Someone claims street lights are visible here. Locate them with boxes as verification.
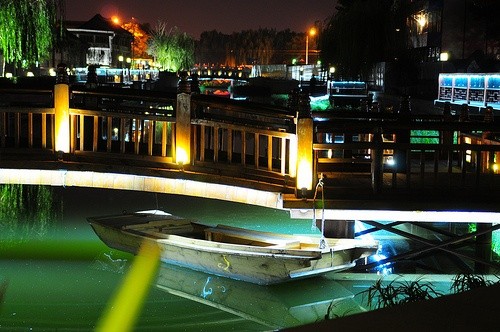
[304,27,316,64]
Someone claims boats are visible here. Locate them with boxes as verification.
[84,209,378,286]
